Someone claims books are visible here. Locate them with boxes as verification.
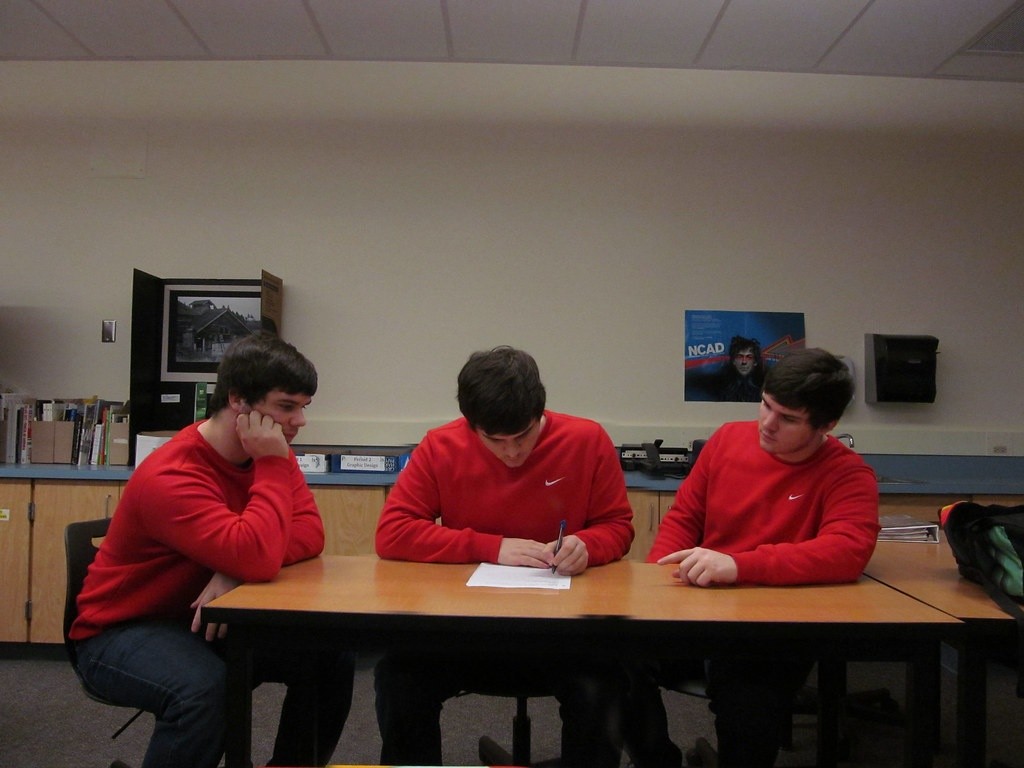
[0,393,132,470]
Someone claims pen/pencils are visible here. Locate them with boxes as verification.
[552,520,567,575]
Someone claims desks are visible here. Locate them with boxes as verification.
[197,554,966,768]
[864,529,1024,768]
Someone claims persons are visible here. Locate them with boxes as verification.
[374,345,638,766]
[615,343,883,768]
[68,328,359,768]
[710,335,777,403]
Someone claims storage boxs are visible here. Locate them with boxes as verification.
[134,431,179,469]
[54,421,75,464]
[330,449,407,475]
[295,450,331,473]
[31,420,54,464]
[108,422,129,466]
[399,449,413,471]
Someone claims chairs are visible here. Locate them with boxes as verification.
[660,670,719,768]
[460,670,562,768]
[63,517,264,768]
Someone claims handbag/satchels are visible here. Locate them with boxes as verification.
[937,501,1024,626]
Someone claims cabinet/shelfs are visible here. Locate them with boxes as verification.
[309,484,441,556]
[26,479,127,660]
[0,477,33,660]
[625,487,677,559]
